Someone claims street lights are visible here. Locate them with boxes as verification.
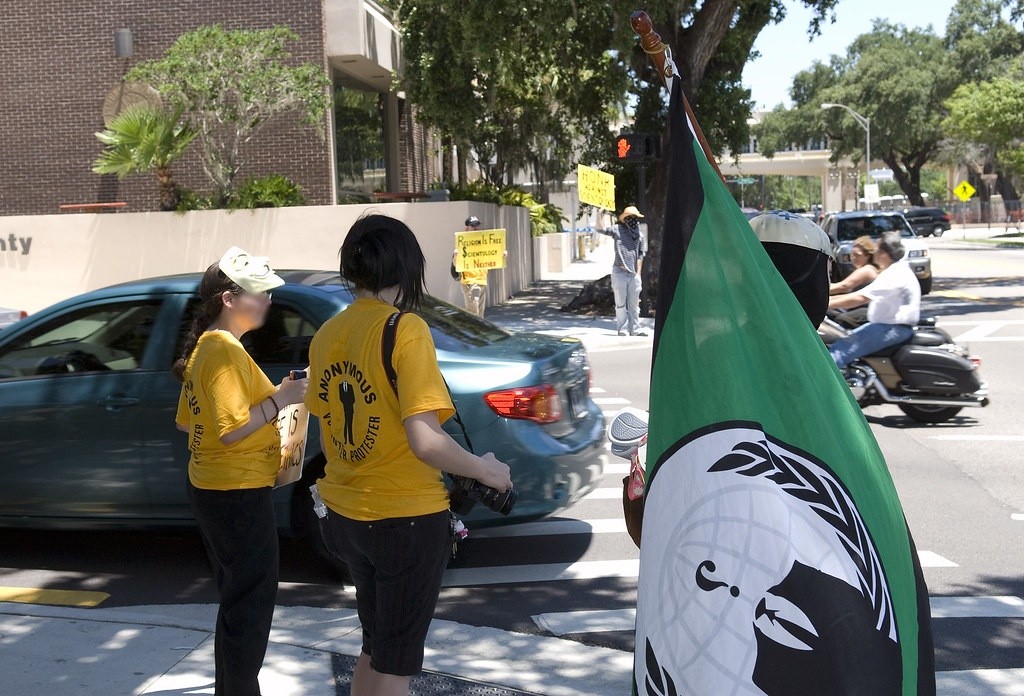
[822,103,873,210]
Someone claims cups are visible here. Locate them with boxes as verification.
[290,370,307,379]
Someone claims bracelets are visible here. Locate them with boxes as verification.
[266,395,279,418]
[260,403,269,425]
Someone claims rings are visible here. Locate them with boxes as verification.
[508,482,513,491]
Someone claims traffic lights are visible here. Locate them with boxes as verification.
[612,133,643,165]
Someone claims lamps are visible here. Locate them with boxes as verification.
[114,29,133,58]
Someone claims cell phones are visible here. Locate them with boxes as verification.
[290,370,307,381]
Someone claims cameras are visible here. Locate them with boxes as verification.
[449,475,520,515]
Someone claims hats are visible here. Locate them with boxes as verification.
[465,216,481,227]
[619,207,646,222]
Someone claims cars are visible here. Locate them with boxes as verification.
[740,208,759,222]
[897,208,951,238]
[0,269,608,579]
[794,213,825,227]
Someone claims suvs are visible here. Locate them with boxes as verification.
[821,210,933,296]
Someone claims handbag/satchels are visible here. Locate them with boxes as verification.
[451,263,463,282]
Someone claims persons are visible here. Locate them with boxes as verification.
[596,206,648,337]
[302,214,513,696]
[825,237,879,329]
[175,249,310,696]
[819,231,921,368]
[453,216,507,321]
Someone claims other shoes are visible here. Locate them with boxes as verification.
[619,330,648,338]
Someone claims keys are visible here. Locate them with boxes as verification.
[451,513,462,557]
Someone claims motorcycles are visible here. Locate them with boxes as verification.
[825,307,953,347]
[817,315,992,423]
